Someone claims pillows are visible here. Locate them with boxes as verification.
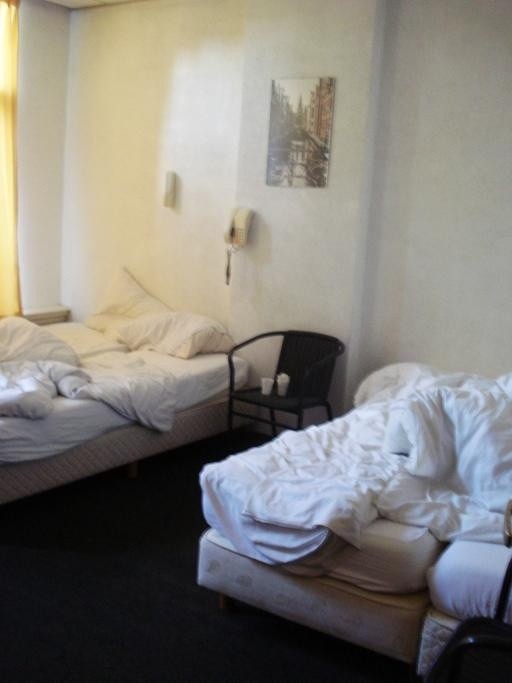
[87,266,235,359]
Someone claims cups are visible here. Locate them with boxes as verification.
[261,377,274,395]
[276,373,290,396]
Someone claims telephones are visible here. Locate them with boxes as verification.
[222,207,254,286]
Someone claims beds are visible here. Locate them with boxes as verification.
[0,265,254,506]
[197,362,512,678]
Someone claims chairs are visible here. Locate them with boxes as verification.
[228,331,346,438]
